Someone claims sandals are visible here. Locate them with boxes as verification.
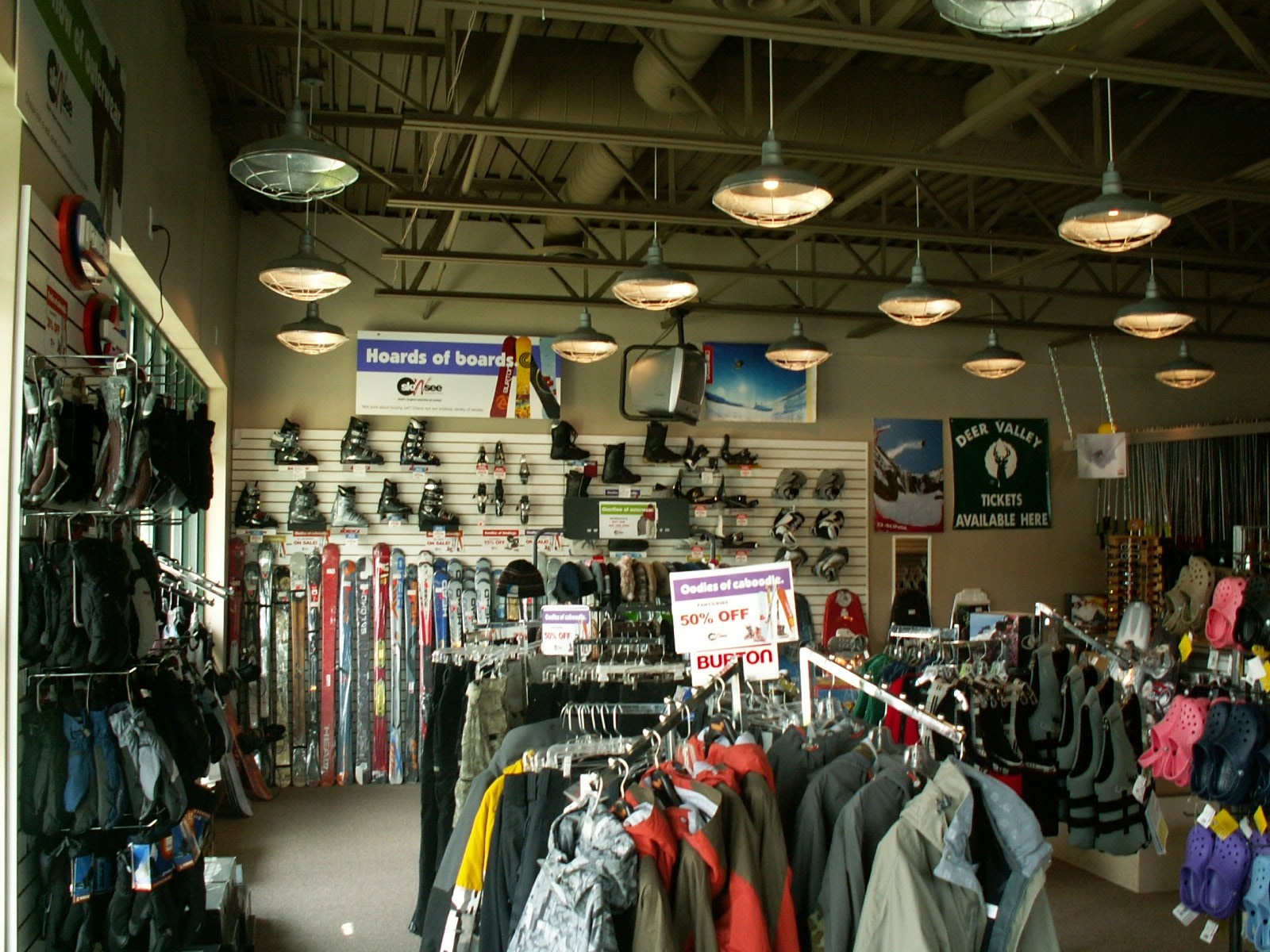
[1137,558,1270,952]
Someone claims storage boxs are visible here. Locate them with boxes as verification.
[203,856,256,952]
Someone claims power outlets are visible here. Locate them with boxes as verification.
[148,203,157,241]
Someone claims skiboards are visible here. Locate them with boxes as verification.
[224,535,561,788]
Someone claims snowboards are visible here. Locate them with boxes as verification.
[489,336,560,418]
[209,659,273,817]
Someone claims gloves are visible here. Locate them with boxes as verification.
[21,364,226,952]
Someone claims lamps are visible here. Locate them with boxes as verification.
[259,202,351,302]
[765,230,830,372]
[1057,78,1171,251]
[1154,261,1217,390]
[879,169,961,326]
[611,148,699,311]
[1114,191,1196,339]
[551,233,619,363]
[230,0,361,202]
[961,231,1026,380]
[276,198,350,355]
[713,39,834,227]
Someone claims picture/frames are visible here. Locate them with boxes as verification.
[890,535,933,628]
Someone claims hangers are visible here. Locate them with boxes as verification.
[158,570,198,612]
[433,604,1270,842]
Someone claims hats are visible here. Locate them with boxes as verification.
[497,560,544,598]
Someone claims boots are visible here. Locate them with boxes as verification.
[331,485,369,527]
[270,418,318,465]
[551,420,589,460]
[418,480,459,532]
[234,481,278,528]
[771,467,850,583]
[399,415,441,466]
[602,444,641,484]
[565,470,592,498]
[341,417,386,464]
[644,421,680,463]
[286,482,328,531]
[377,480,411,514]
